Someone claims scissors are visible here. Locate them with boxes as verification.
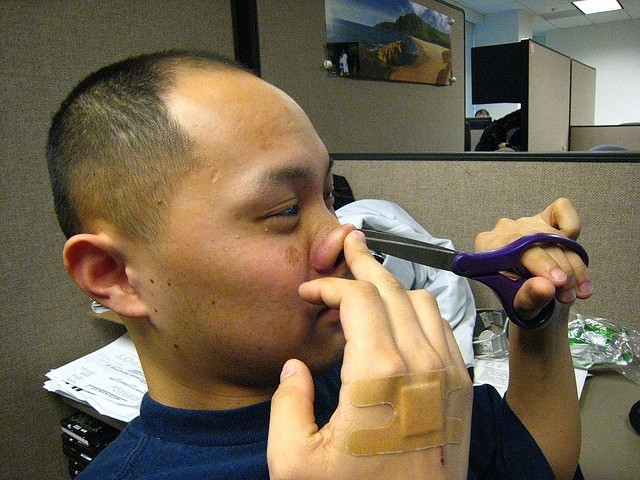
[357,229,589,330]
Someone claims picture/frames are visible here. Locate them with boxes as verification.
[323,0,456,87]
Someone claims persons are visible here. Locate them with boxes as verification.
[45,49,594,479]
[343,49,348,74]
[474,109,489,117]
[339,53,343,76]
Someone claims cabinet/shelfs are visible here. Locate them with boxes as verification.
[43,310,126,478]
[471,39,596,153]
